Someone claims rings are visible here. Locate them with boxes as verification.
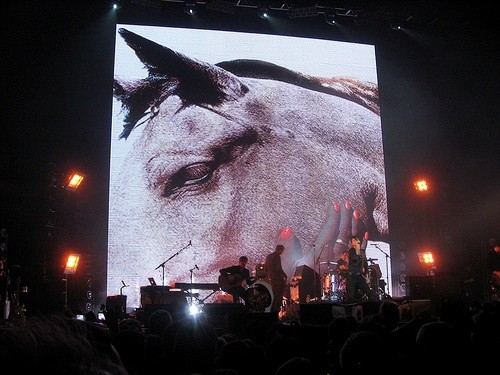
[336,239,347,245]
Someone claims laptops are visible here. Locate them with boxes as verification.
[148,278,156,285]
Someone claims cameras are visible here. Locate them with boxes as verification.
[77,315,86,321]
[98,313,106,320]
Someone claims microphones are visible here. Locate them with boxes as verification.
[195,265,199,270]
[370,244,377,245]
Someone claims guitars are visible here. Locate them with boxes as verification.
[219,272,259,291]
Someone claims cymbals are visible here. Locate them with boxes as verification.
[367,259,378,261]
[319,261,338,265]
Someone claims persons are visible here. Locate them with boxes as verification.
[219,256,251,303]
[337,251,349,271]
[278,200,369,285]
[346,236,369,303]
[264,244,287,312]
[0,300,500,375]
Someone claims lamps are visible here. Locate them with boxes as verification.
[58,250,81,272]
[62,166,84,189]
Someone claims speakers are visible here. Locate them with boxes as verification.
[105,296,126,330]
[205,303,243,336]
[289,264,322,302]
[228,312,278,340]
[294,303,333,325]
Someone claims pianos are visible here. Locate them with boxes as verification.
[170,282,219,316]
